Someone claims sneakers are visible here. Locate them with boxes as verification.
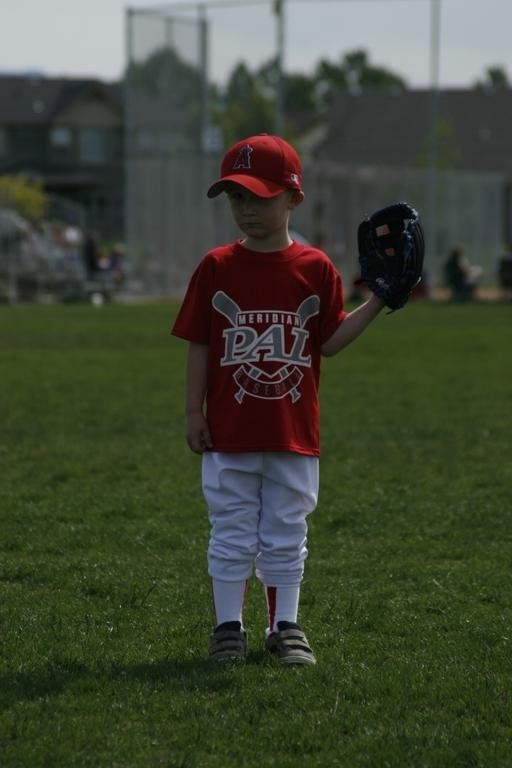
[209,620,248,672]
[264,620,316,667]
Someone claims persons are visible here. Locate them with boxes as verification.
[444,245,474,306]
[170,132,427,670]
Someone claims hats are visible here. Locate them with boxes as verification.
[206,132,303,199]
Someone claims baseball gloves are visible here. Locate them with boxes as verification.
[354,202,425,315]
